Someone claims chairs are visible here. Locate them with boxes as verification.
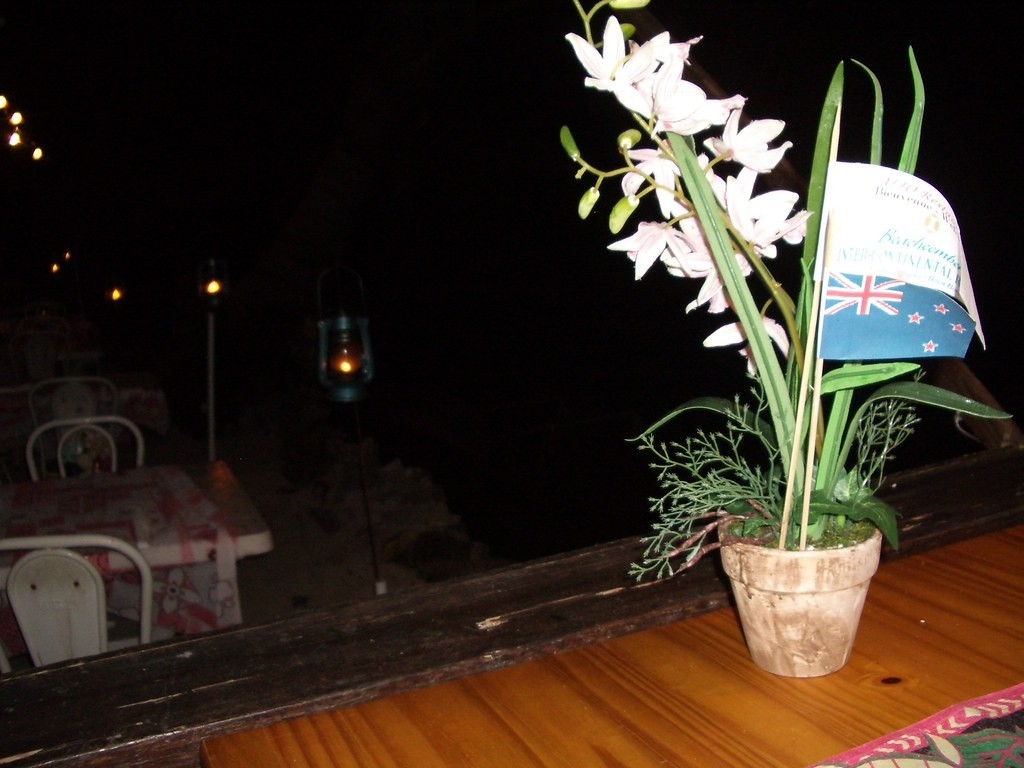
[0,532,152,673]
[7,300,145,481]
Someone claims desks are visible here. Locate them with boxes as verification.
[0,371,170,475]
[0,460,272,636]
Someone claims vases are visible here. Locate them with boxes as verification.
[717,508,882,677]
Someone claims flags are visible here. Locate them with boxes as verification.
[813,162,986,359]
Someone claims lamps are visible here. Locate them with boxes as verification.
[316,309,382,600]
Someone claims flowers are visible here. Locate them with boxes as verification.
[560,0,1014,583]
